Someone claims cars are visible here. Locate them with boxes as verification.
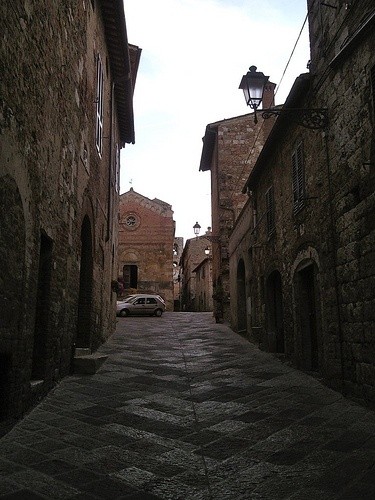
[115,295,165,317]
[116,294,147,305]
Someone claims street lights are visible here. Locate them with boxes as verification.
[237,65,348,398]
[194,222,222,272]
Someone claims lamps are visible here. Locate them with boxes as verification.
[193,222,219,243]
[239,66,327,129]
[204,246,210,254]
